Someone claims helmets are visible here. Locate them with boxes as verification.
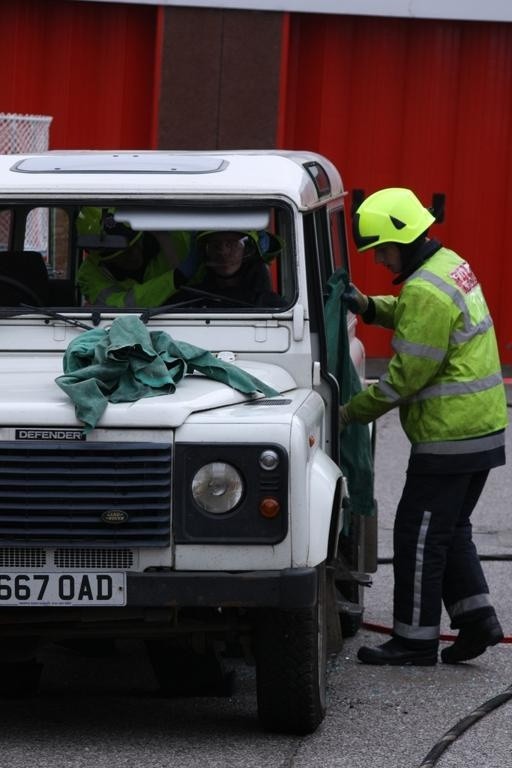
[352,187,437,254]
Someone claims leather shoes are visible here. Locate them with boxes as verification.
[441,614,504,664]
[357,636,439,664]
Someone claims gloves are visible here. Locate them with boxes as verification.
[343,282,368,315]
[339,405,351,436]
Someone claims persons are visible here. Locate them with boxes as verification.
[77,204,289,308]
[339,188,509,667]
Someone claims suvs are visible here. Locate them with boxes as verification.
[0,150,380,736]
